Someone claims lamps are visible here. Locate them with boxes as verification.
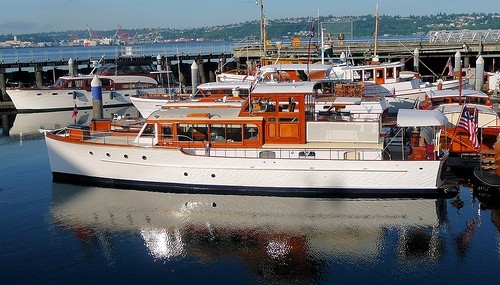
[248,128,256,137]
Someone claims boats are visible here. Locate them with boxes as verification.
[0,71,182,137]
[37,75,450,189]
[196,0,500,160]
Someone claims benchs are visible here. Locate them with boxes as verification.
[91,118,111,132]
[67,124,91,140]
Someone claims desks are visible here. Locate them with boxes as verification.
[192,131,205,140]
